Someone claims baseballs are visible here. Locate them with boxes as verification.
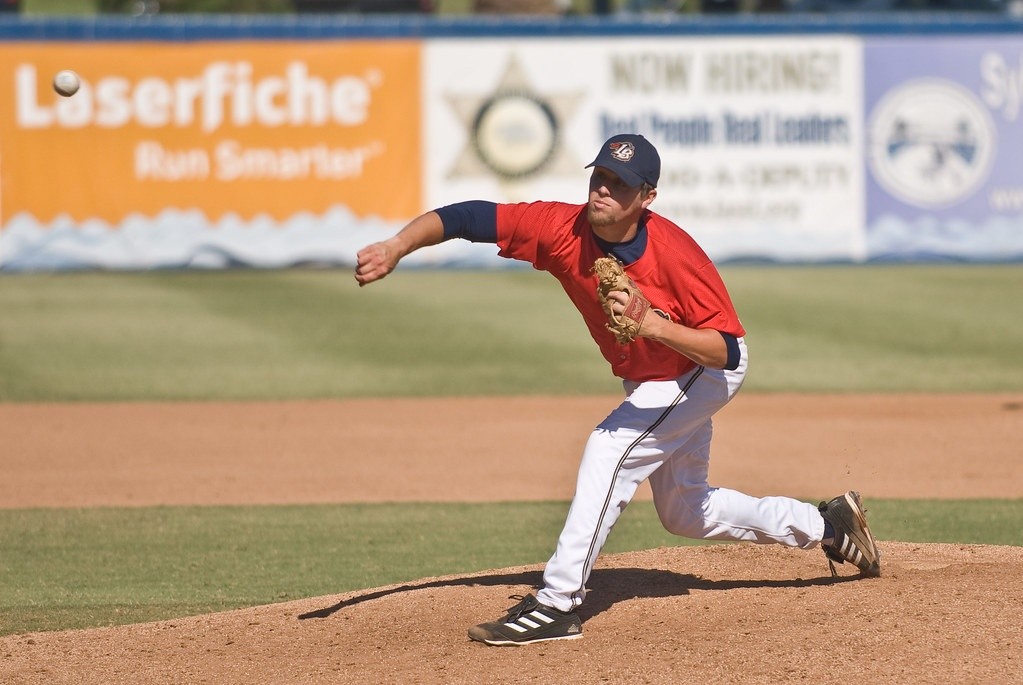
[53,70,80,97]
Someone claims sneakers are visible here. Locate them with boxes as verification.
[467,592,585,646]
[818,489,881,577]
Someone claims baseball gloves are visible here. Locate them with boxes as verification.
[588,252,651,345]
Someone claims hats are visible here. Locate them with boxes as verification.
[585,133,661,188]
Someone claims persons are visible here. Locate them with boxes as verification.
[354,132,879,647]
